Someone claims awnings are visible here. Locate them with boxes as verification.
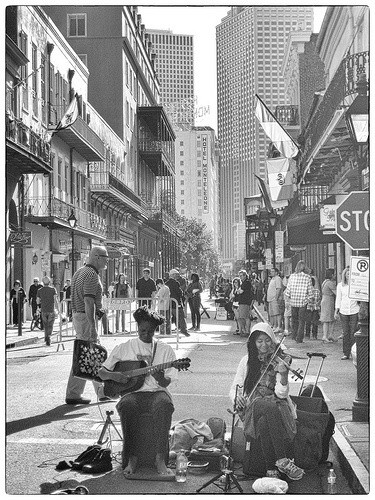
[286,212,342,244]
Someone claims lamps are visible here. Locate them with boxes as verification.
[33,252,38,264]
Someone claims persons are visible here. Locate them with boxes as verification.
[112,274,129,333]
[136,268,202,337]
[334,268,361,360]
[63,279,73,322]
[267,265,321,339]
[283,260,315,343]
[229,270,265,337]
[209,273,232,299]
[28,278,43,330]
[230,322,304,480]
[10,279,26,325]
[65,245,110,404]
[98,304,178,476]
[319,268,338,343]
[102,309,112,334]
[36,277,56,346]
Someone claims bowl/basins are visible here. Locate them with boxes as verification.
[187,461,209,474]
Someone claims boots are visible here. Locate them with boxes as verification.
[71,444,102,470]
[83,448,113,473]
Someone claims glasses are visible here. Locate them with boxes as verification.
[33,280,38,282]
[94,253,110,258]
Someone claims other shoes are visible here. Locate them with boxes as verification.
[14,322,23,327]
[66,397,92,404]
[45,337,50,346]
[181,326,201,337]
[291,334,318,343]
[104,329,129,334]
[271,325,291,337]
[341,356,348,360]
[233,329,249,338]
[99,396,111,401]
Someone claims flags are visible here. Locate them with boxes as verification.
[255,100,298,159]
[62,96,80,129]
[266,158,298,200]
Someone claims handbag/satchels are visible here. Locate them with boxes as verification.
[71,338,108,383]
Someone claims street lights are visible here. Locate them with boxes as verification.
[67,208,77,276]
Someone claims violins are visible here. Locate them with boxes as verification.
[264,343,304,381]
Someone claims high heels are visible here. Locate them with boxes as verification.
[321,336,339,345]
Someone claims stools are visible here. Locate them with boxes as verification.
[243,437,294,477]
[122,413,170,468]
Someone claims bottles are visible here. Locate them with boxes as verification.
[175,449,188,483]
[327,469,338,494]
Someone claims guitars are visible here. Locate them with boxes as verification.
[103,356,191,399]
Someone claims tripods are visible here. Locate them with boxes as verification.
[98,411,122,444]
[194,383,244,494]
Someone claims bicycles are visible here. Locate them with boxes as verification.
[30,303,44,331]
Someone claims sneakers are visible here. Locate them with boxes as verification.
[275,458,306,480]
[267,470,282,480]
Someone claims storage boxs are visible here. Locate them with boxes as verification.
[188,440,227,474]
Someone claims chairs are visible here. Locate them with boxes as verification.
[200,304,210,318]
[214,285,232,320]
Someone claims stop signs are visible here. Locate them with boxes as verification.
[333,191,369,251]
